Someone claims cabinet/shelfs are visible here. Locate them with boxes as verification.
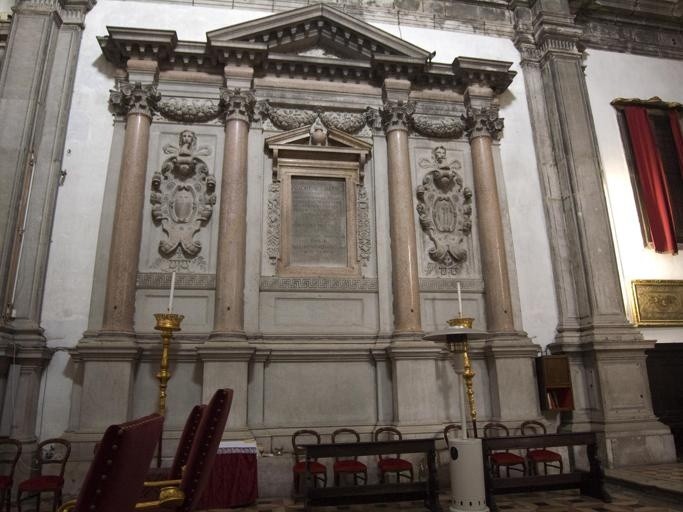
[534,354,574,411]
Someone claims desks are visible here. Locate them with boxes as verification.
[479,431,615,511]
[201,439,258,508]
[297,435,446,512]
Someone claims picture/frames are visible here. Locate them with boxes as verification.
[629,278,683,328]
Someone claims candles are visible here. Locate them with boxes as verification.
[167,271,175,311]
[456,280,463,318]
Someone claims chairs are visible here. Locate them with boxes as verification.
[442,422,564,477]
[59,385,237,511]
[288,426,415,508]
[0,430,69,511]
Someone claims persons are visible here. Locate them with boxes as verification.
[178,129,200,153]
[430,146,447,166]
